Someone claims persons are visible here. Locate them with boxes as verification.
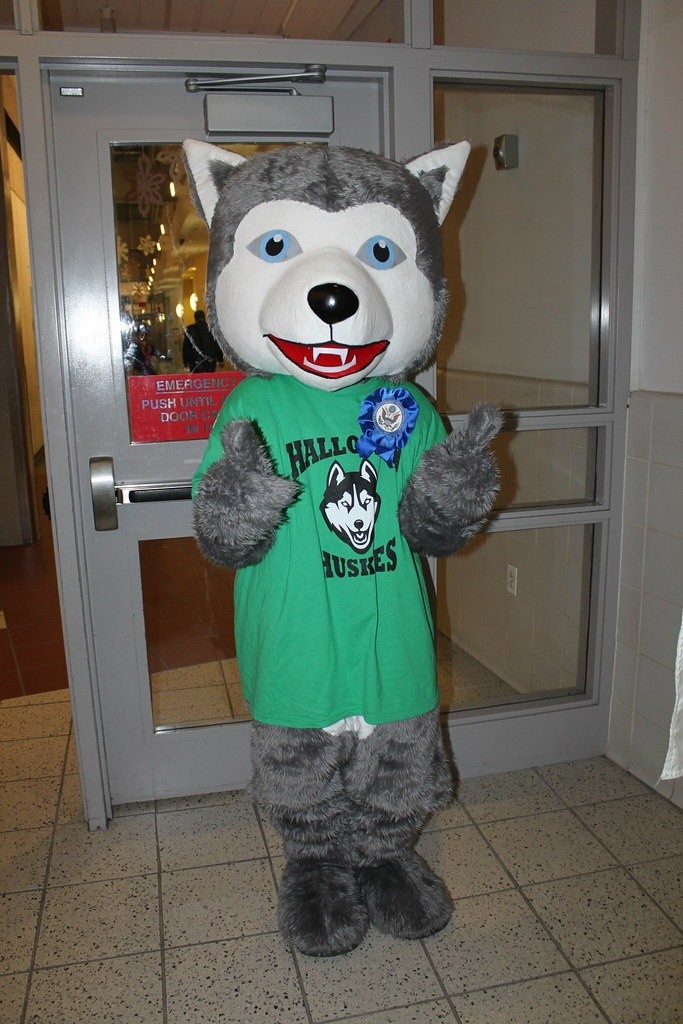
[182,310,224,372]
[123,322,159,376]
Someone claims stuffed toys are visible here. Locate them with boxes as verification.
[180,137,509,956]
